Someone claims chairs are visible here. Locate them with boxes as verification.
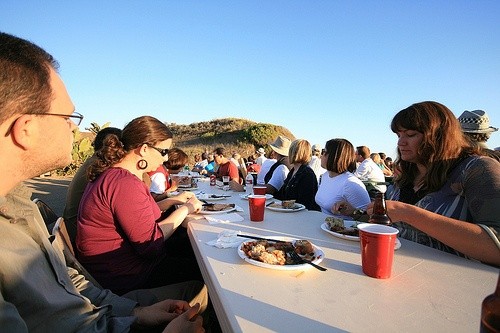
[33,197,105,288]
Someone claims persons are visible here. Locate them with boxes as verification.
[330,100,500,266]
[74,116,203,284]
[0,31,205,332]
[63,126,394,247]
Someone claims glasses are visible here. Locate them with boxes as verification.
[6,112,83,136]
[149,145,168,157]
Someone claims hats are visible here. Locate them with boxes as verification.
[269,135,291,156]
[256,148,266,154]
[457,110,498,134]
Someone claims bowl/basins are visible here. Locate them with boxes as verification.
[253,188,267,195]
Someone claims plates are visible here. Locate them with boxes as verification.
[197,194,231,201]
[321,220,367,241]
[176,184,199,190]
[197,203,236,214]
[265,201,307,212]
[237,236,325,270]
[241,194,274,199]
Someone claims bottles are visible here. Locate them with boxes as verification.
[369,192,393,227]
[479,271,500,333]
[210,173,217,186]
[245,172,255,196]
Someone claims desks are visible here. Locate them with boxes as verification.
[171,176,309,228]
[187,210,500,333]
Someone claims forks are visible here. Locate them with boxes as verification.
[290,250,328,271]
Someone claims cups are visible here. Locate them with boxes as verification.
[247,195,267,221]
[357,223,400,279]
[222,176,230,185]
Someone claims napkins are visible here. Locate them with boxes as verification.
[204,229,250,249]
[202,211,244,225]
